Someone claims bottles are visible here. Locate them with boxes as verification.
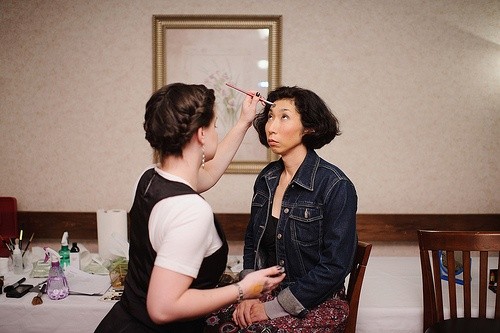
[490,272,496,282]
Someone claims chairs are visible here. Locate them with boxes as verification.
[343,241,372,333]
[417,229,500,333]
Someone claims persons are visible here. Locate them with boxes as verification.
[206,86,358,333]
[93,84,286,333]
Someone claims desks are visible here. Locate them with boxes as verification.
[0,255,243,333]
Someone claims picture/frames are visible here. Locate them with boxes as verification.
[151,12,284,174]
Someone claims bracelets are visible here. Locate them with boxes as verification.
[233,283,243,303]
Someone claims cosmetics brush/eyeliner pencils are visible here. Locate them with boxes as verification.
[2,230,46,304]
[225,84,275,107]
